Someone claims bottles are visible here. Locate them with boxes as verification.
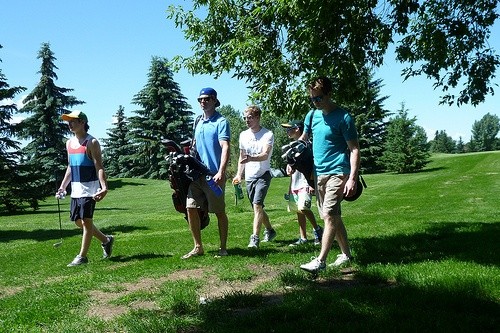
[234,180,244,199]
[205,175,223,196]
[59,188,65,202]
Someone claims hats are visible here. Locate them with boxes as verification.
[61,110,88,122]
[281,120,305,128]
[199,88,220,108]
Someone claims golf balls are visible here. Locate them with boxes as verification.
[55,190,67,200]
[92,188,101,198]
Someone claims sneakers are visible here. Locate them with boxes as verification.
[327,252,354,267]
[289,238,308,247]
[261,228,276,243]
[67,255,88,269]
[248,234,261,250]
[300,257,327,274]
[313,226,324,246]
[101,235,114,258]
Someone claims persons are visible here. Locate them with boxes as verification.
[282,121,324,246]
[55,111,114,268]
[181,88,231,259]
[232,105,276,249]
[296,76,360,274]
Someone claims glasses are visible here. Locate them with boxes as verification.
[310,94,326,102]
[243,115,257,121]
[198,97,213,102]
[286,127,299,131]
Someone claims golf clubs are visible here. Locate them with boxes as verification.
[53,169,63,247]
[160,139,197,176]
[280,139,306,161]
